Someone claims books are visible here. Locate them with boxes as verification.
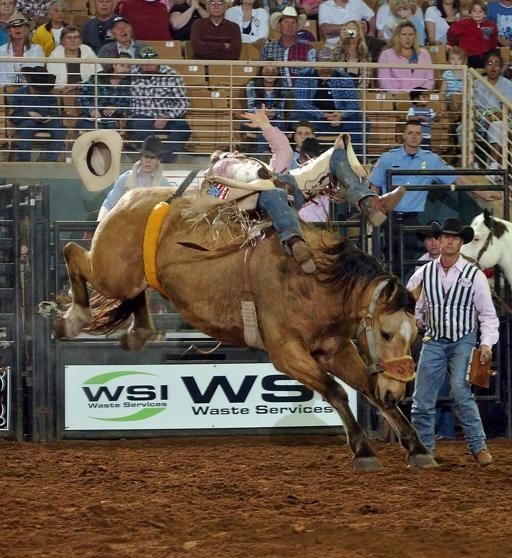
[466,347,494,389]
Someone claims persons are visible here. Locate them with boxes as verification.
[407,218,499,469]
[416,221,459,441]
[293,46,371,165]
[0,0,16,45]
[318,20,370,90]
[116,46,192,163]
[376,1,425,47]
[241,56,292,155]
[318,0,387,63]
[255,7,316,119]
[447,2,502,69]
[440,48,468,97]
[0,11,46,86]
[113,0,169,41]
[368,121,503,286]
[378,21,435,93]
[204,106,405,274]
[46,26,104,92]
[81,1,120,55]
[9,65,67,162]
[32,1,71,57]
[425,1,465,46]
[225,1,269,44]
[393,2,427,47]
[406,86,436,148]
[191,0,242,61]
[98,17,148,71]
[74,53,132,132]
[486,0,511,40]
[97,135,169,222]
[169,0,209,40]
[450,49,512,180]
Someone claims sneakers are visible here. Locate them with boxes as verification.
[486,173,501,182]
[474,161,479,167]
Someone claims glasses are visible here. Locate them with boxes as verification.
[488,59,499,66]
[206,0,227,6]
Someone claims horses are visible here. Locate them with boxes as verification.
[458,208,511,287]
[51,188,442,473]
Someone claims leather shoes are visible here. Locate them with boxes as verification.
[476,450,495,468]
[358,186,406,228]
[288,237,318,275]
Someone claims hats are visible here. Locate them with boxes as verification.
[432,217,476,245]
[136,135,166,158]
[316,46,336,61]
[138,47,161,58]
[21,64,58,92]
[5,10,28,28]
[106,13,129,29]
[268,7,310,30]
[415,219,442,238]
